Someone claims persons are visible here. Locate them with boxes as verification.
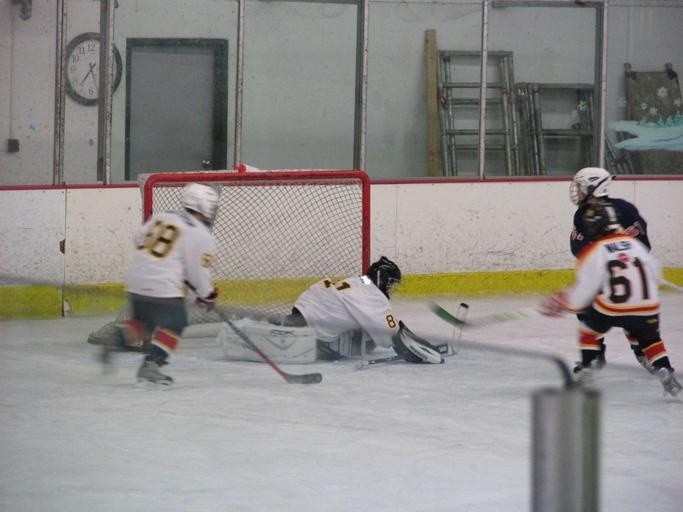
[565,167,662,376]
[540,200,680,400]
[83,180,219,389]
[216,250,458,365]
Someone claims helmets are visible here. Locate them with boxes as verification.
[569,167,613,206]
[366,256,401,298]
[180,181,221,226]
[575,206,610,239]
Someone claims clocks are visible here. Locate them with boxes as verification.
[64,32,122,105]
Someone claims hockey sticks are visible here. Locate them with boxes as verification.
[353,302,468,369]
[429,303,540,328]
[182,282,322,384]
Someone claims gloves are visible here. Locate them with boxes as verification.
[195,288,219,312]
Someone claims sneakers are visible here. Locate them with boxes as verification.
[136,360,172,387]
[572,355,606,375]
[660,372,682,399]
